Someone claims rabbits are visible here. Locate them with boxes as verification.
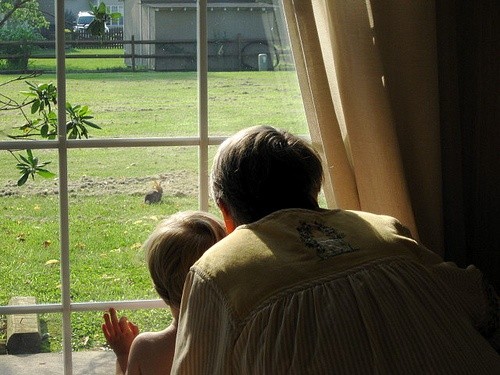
[144,180,163,206]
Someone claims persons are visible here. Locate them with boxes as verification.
[169,122,500,375]
[102,210,229,375]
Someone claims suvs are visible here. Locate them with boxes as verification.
[73,11,110,39]
